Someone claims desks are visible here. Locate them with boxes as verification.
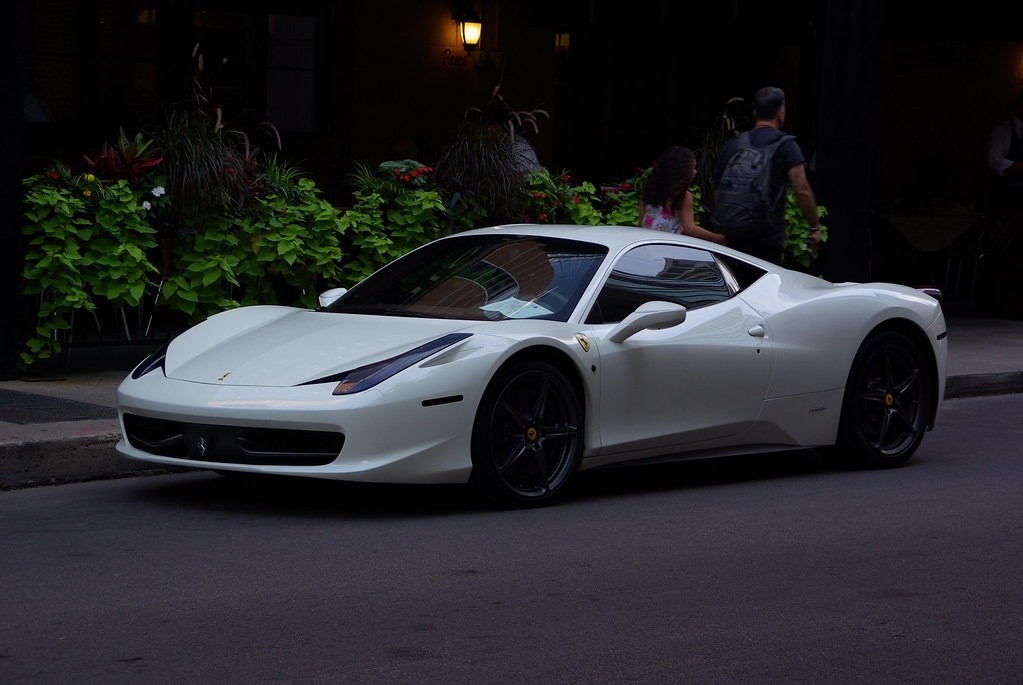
[881,210,978,294]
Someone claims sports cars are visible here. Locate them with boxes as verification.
[115,222,949,509]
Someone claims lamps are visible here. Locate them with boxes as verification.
[451,5,483,57]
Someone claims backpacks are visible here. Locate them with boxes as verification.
[710,132,796,233]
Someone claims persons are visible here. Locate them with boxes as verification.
[714,87,821,268]
[392,124,540,226]
[638,146,726,245]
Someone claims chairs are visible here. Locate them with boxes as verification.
[944,227,992,297]
